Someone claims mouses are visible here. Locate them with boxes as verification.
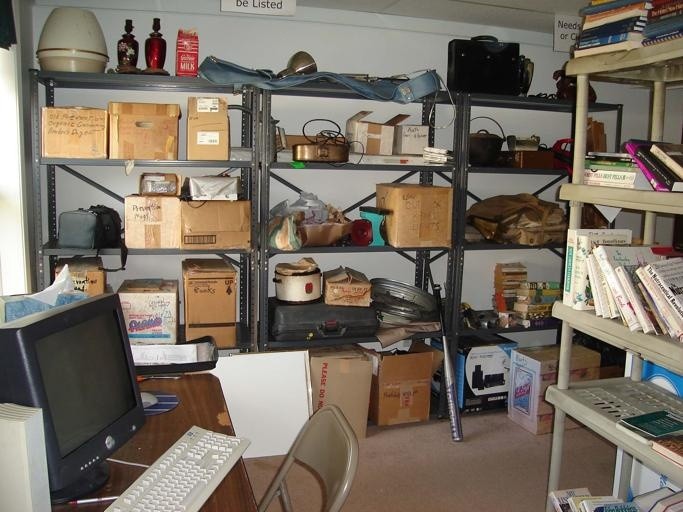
[140,391,158,408]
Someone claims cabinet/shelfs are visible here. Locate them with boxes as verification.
[544,36,682,512]
[28,67,260,354]
[260,79,463,422]
[446,92,624,418]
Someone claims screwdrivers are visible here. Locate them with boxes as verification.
[136,374,181,382]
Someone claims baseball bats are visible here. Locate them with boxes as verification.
[432,284,463,440]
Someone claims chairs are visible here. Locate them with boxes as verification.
[258,405,359,512]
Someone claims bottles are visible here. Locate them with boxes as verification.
[115,19,139,69]
[144,17,166,70]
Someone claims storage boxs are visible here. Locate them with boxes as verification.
[375,183,454,248]
[507,343,601,436]
[322,266,371,308]
[41,104,108,160]
[343,110,411,156]
[420,333,519,417]
[124,173,252,251]
[362,341,444,426]
[187,96,231,161]
[393,124,430,156]
[270,345,373,441]
[54,258,238,349]
[108,101,182,161]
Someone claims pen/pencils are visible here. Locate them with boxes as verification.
[67,496,119,505]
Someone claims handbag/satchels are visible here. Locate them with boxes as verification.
[448,35,520,96]
[58,207,120,249]
[466,191,566,244]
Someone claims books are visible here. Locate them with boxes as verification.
[563,229,683,341]
[583,139,683,193]
[549,487,683,512]
[617,407,683,466]
[493,262,563,328]
[574,0,683,57]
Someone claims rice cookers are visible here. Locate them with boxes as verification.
[273,269,323,305]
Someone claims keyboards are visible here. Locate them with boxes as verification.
[104,425,251,512]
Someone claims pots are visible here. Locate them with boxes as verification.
[292,142,351,163]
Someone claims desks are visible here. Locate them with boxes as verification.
[50,372,261,512]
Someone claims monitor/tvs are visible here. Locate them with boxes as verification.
[0,294,148,504]
